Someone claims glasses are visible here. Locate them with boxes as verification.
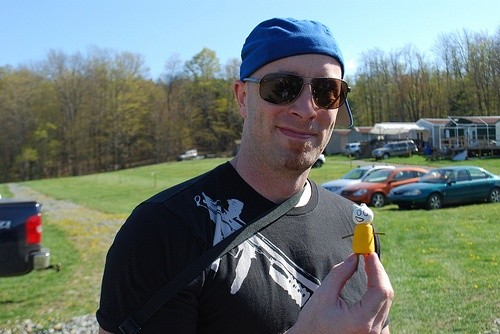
[242,73,352,110]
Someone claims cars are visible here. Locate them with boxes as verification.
[387,166,500,210]
[320,165,403,195]
[0,201,50,278]
[312,154,326,168]
[341,166,448,208]
[177,149,198,161]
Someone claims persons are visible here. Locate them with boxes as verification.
[95,16,395,334]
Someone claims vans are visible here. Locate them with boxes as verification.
[344,142,361,155]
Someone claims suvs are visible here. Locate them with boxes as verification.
[371,140,418,160]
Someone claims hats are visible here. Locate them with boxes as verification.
[240,18,345,82]
